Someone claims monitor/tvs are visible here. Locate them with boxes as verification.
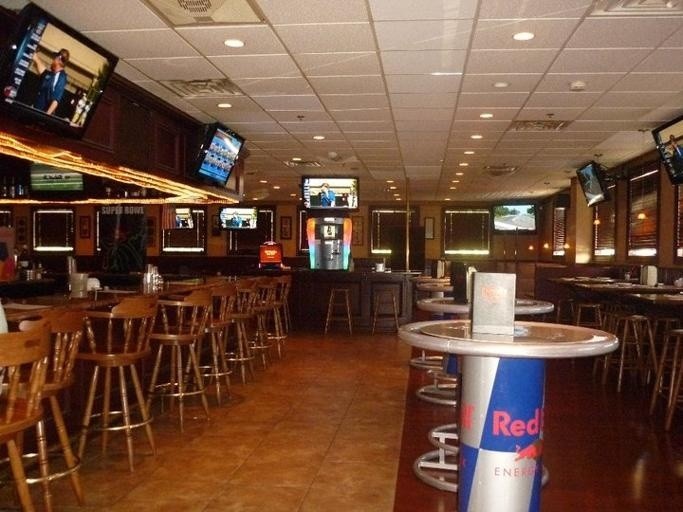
[28,160,86,199]
[175,207,195,230]
[302,175,359,212]
[490,203,537,235]
[191,122,246,186]
[0,1,120,142]
[575,160,610,208]
[218,206,259,231]
[650,115,683,186]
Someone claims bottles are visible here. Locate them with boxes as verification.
[1,171,25,197]
[142,263,163,286]
[103,180,146,197]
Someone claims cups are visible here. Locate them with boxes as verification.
[375,262,385,273]
[68,273,89,298]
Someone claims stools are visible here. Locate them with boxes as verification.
[322,286,354,336]
[370,287,399,336]
